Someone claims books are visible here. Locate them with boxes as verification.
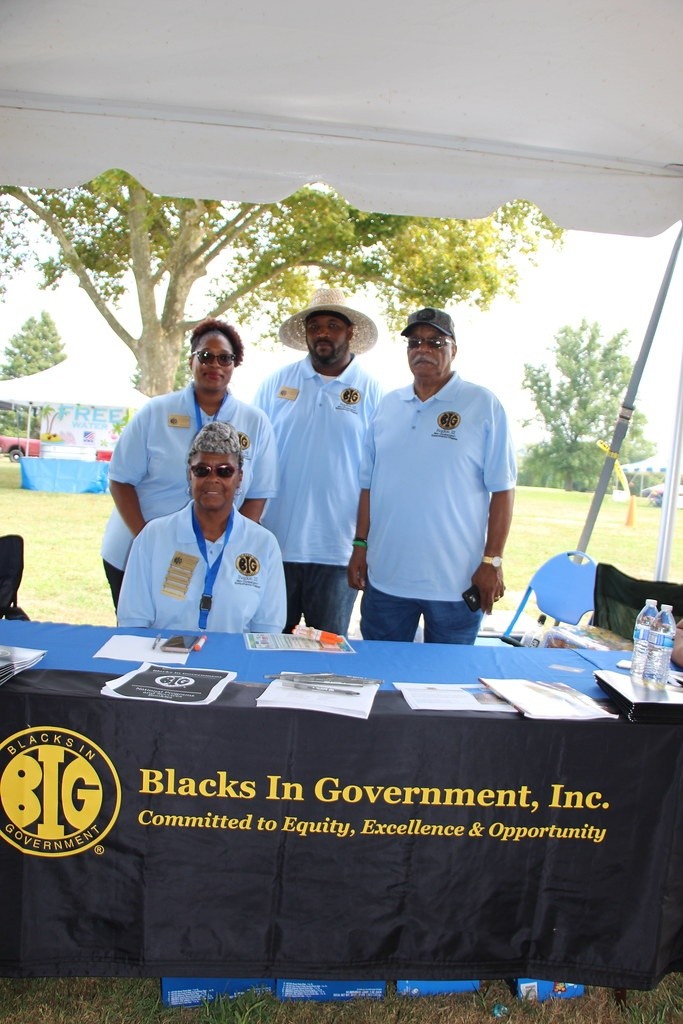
[159,633,200,653]
[100,662,237,705]
[477,678,619,722]
[593,670,683,725]
[0,644,48,685]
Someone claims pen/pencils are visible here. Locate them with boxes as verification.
[152,633,162,649]
[264,672,384,688]
[282,682,360,696]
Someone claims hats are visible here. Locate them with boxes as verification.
[279,288,378,355]
[401,308,456,340]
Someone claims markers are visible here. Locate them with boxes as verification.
[291,625,343,645]
[195,635,207,651]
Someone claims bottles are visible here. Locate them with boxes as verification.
[520,614,547,647]
[630,600,659,676]
[643,604,676,684]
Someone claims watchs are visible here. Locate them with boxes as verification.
[482,555,503,568]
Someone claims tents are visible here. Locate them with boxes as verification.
[0,338,154,457]
[614,453,683,509]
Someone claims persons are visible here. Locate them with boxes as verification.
[670,618,683,669]
[102,320,278,615]
[254,289,382,635]
[347,308,516,645]
[117,422,287,633]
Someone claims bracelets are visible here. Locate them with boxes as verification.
[352,537,368,550]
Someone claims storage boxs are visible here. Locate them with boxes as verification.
[512,978,584,1002]
[544,623,634,651]
[397,980,480,997]
[162,975,277,1008]
[276,978,386,1001]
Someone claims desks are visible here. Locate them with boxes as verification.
[20,457,110,493]
[0,619,683,1011]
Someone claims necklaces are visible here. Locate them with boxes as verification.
[192,499,234,629]
[192,380,228,432]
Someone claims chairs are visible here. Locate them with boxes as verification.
[476,551,601,647]
[0,534,31,621]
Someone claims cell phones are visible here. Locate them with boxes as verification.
[161,635,201,653]
[462,585,481,612]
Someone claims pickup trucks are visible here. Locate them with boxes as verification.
[0,435,40,462]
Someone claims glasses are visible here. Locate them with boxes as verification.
[189,462,236,478]
[405,335,453,349]
[192,351,236,367]
